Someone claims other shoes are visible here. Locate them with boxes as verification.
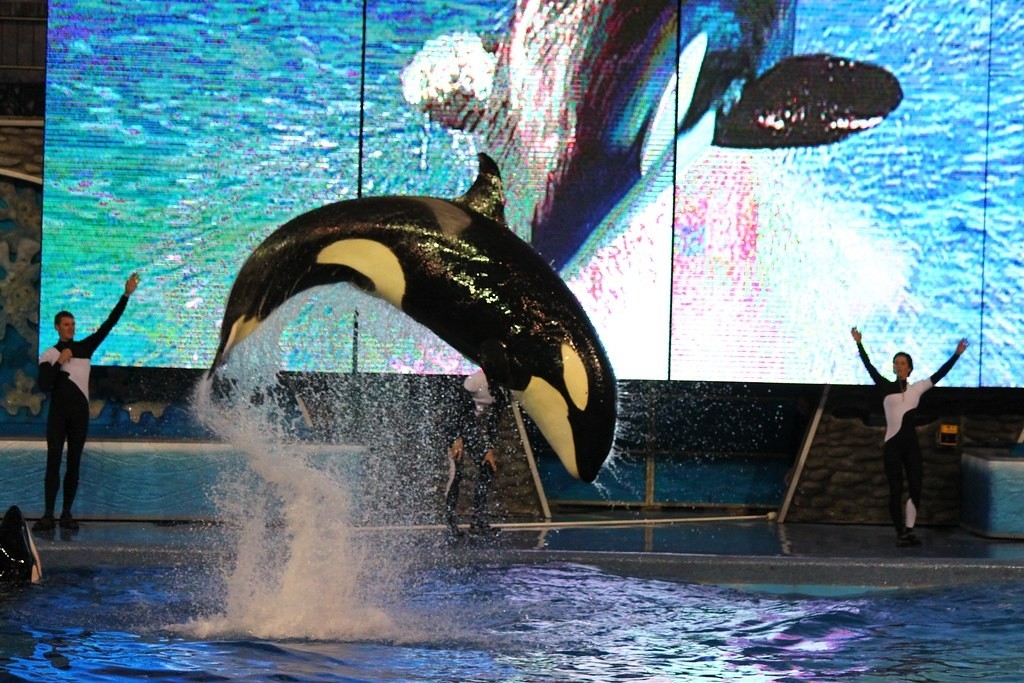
[444,525,465,536]
[32,512,56,531]
[468,524,500,536]
[897,532,922,549]
[61,514,79,531]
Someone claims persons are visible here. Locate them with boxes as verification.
[436,383,502,535]
[31,270,140,538]
[851,327,967,549]
